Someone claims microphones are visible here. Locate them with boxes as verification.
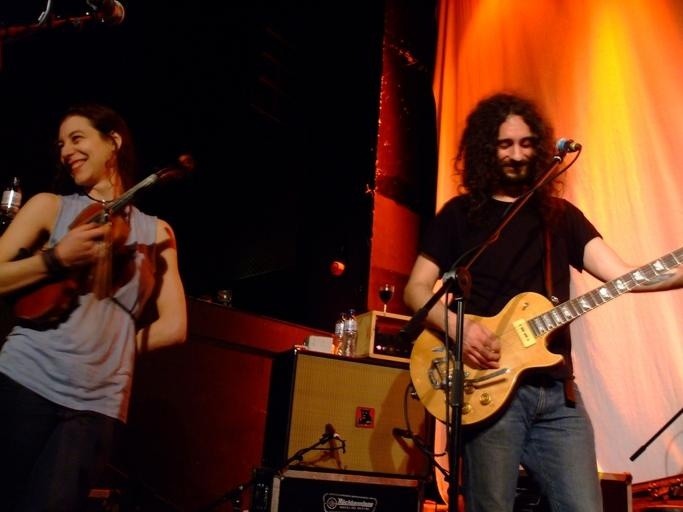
[553,137,581,153]
[88,0,125,27]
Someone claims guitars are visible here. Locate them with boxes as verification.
[409,247,682,426]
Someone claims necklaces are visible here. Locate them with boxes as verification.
[86,192,114,206]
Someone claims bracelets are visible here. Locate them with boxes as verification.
[41,246,63,275]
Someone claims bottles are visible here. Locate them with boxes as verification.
[332,307,357,358]
[1,173,23,232]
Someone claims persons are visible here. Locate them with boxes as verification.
[403,93,683,511]
[0,102,189,512]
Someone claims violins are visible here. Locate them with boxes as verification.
[2,153,195,326]
